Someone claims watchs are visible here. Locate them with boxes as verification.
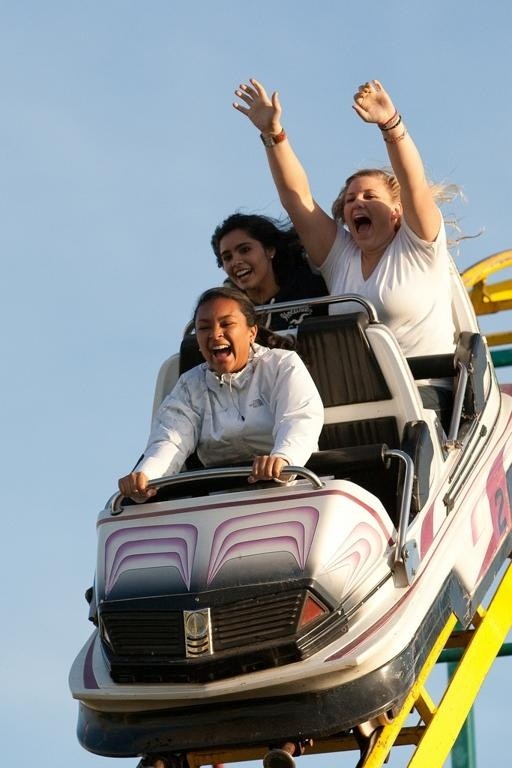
[257,125,291,151]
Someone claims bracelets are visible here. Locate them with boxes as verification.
[376,107,409,146]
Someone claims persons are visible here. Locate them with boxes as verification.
[230,76,482,428]
[210,211,331,332]
[115,287,324,505]
